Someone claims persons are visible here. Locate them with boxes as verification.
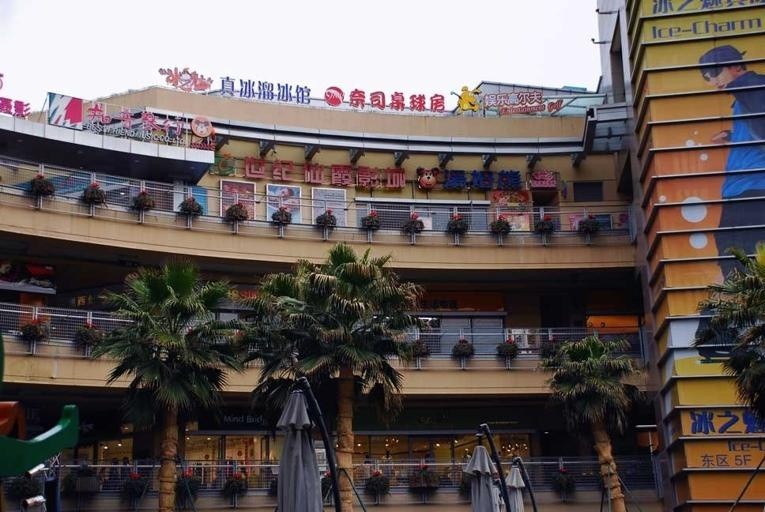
[270,185,300,213]
[698,45,765,280]
[461,448,471,463]
[121,456,131,480]
[0,263,16,279]
[381,450,393,463]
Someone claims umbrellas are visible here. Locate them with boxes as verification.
[501,456,537,511]
[275,374,344,512]
[467,421,510,512]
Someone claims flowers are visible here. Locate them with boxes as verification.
[18,317,51,345]
[450,339,476,358]
[540,333,561,364]
[406,337,431,358]
[495,336,520,359]
[73,321,106,349]
